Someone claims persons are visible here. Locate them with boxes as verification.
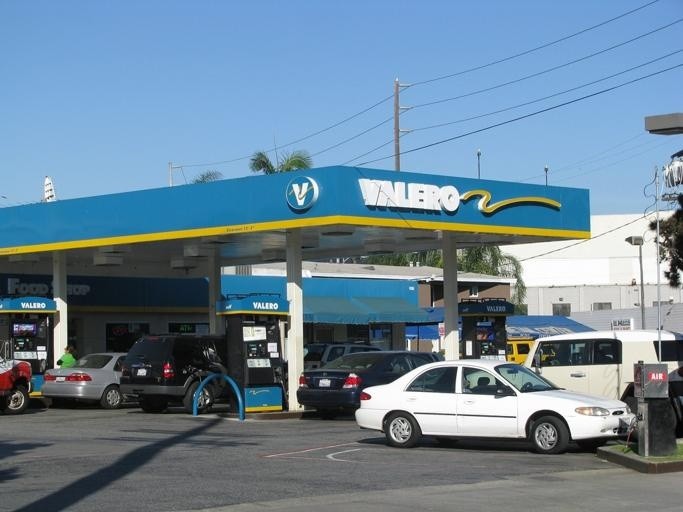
[57,345,77,367]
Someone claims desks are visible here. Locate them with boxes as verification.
[626,236,645,332]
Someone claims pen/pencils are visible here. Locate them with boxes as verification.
[506,338,556,366]
[522,331,682,442]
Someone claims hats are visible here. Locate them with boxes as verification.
[297,350,441,418]
[43,353,129,409]
[0,355,32,414]
[355,360,636,455]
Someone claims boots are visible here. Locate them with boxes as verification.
[304,342,381,373]
[120,334,229,414]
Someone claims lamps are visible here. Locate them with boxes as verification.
[470,377,508,394]
